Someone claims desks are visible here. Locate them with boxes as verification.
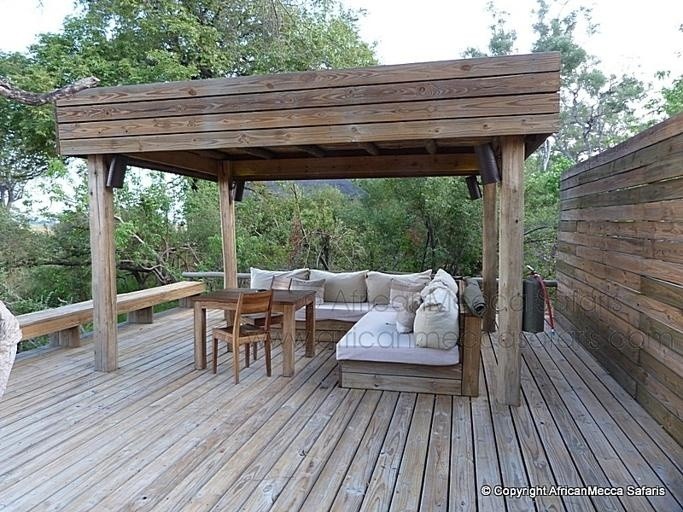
[192,288,316,377]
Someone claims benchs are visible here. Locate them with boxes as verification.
[237,262,486,397]
[13,278,203,349]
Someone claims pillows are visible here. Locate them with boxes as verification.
[248,266,460,351]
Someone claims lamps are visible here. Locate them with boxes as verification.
[475,142,501,184]
[465,176,484,201]
[106,156,126,189]
[230,179,245,202]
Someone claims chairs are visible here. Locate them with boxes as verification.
[210,289,272,385]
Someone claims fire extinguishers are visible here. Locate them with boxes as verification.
[522,265,555,334]
